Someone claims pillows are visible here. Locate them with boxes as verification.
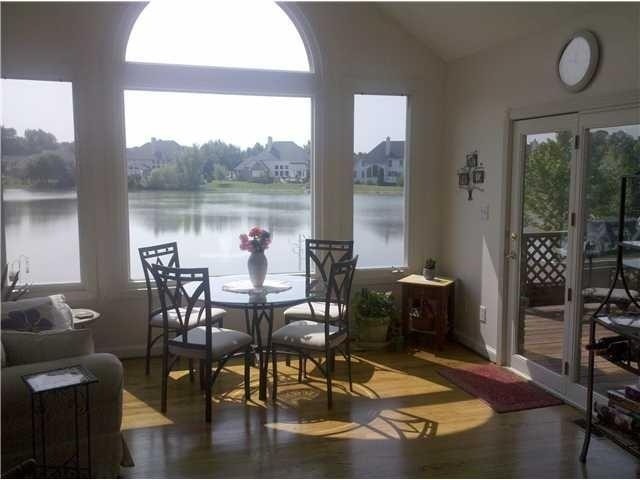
[1,292,75,331]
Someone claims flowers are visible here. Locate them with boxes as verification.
[239,228,274,253]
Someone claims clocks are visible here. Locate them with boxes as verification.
[553,28,602,93]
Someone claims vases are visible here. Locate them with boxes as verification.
[248,252,268,288]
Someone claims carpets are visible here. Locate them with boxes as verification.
[437,360,562,415]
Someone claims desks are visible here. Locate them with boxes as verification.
[71,306,101,329]
[178,273,330,402]
[20,361,100,479]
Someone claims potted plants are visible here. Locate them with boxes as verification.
[353,285,396,341]
[422,256,438,280]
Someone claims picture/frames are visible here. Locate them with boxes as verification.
[465,153,479,167]
[472,170,486,183]
[458,174,470,185]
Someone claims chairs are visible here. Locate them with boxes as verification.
[150,262,252,424]
[577,239,637,346]
[139,240,226,375]
[284,238,353,373]
[270,253,361,410]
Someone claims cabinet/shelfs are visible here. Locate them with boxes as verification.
[398,273,456,354]
[577,176,639,466]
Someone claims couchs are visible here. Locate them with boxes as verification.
[1,327,126,479]
